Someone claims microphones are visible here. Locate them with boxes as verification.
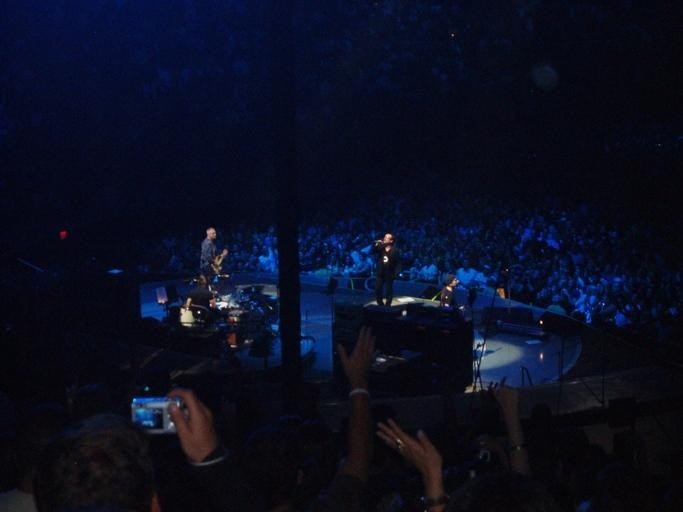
[373,240,384,243]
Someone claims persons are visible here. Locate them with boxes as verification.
[1,176,682,512]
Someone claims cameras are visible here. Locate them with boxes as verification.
[127,396,183,437]
